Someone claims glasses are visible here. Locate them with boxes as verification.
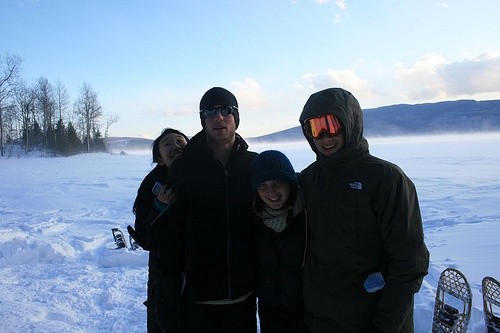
[200,106,238,118]
[303,113,345,140]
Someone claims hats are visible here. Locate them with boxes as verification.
[199,87,239,130]
[248,150,297,188]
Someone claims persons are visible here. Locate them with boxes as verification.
[251,150,310,333]
[127,128,190,333]
[299,88,429,333]
[172,88,260,333]
